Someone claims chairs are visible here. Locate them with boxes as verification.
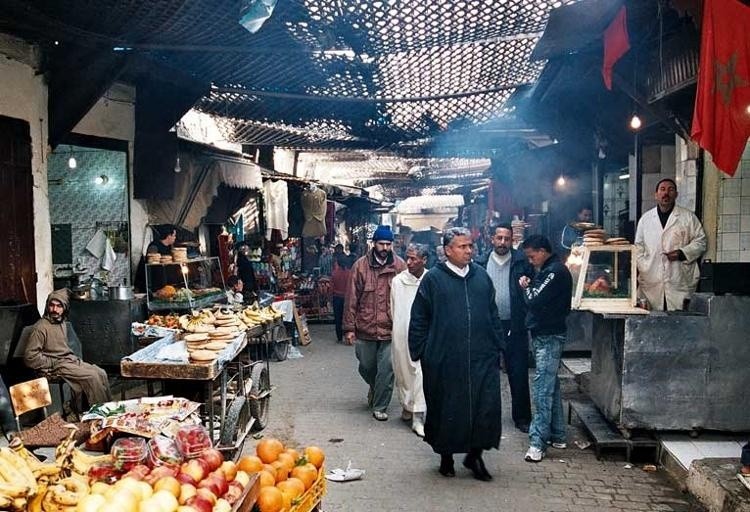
[9,377,78,459]
[14,321,83,421]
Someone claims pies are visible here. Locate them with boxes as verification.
[184,315,244,364]
[146,247,189,264]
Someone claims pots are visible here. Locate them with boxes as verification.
[107,284,136,300]
[72,274,103,300]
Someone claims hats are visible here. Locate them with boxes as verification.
[372,224,394,242]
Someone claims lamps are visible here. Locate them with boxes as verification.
[173,149,182,173]
[630,39,642,129]
[95,175,108,185]
[557,132,567,186]
[68,146,77,168]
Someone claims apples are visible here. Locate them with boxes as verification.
[74,449,249,512]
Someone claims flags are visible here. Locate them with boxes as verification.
[691,0,750,177]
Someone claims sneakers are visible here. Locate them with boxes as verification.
[372,412,388,421]
[520,424,530,433]
[524,446,545,463]
[403,411,411,420]
[412,421,425,436]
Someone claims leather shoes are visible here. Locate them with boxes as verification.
[439,459,456,477]
[463,453,493,481]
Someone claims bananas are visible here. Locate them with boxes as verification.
[179,309,215,332]
[0,435,89,511]
[243,304,280,332]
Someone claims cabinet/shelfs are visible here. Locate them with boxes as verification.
[294,292,336,321]
[563,243,638,312]
[145,257,227,318]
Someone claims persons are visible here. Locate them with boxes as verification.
[394,239,535,372]
[390,243,429,438]
[518,234,573,462]
[474,223,535,433]
[330,243,360,343]
[24,287,113,414]
[236,245,256,305]
[408,227,508,480]
[223,275,244,305]
[145,224,178,315]
[0,373,21,448]
[343,225,407,422]
[633,179,707,311]
